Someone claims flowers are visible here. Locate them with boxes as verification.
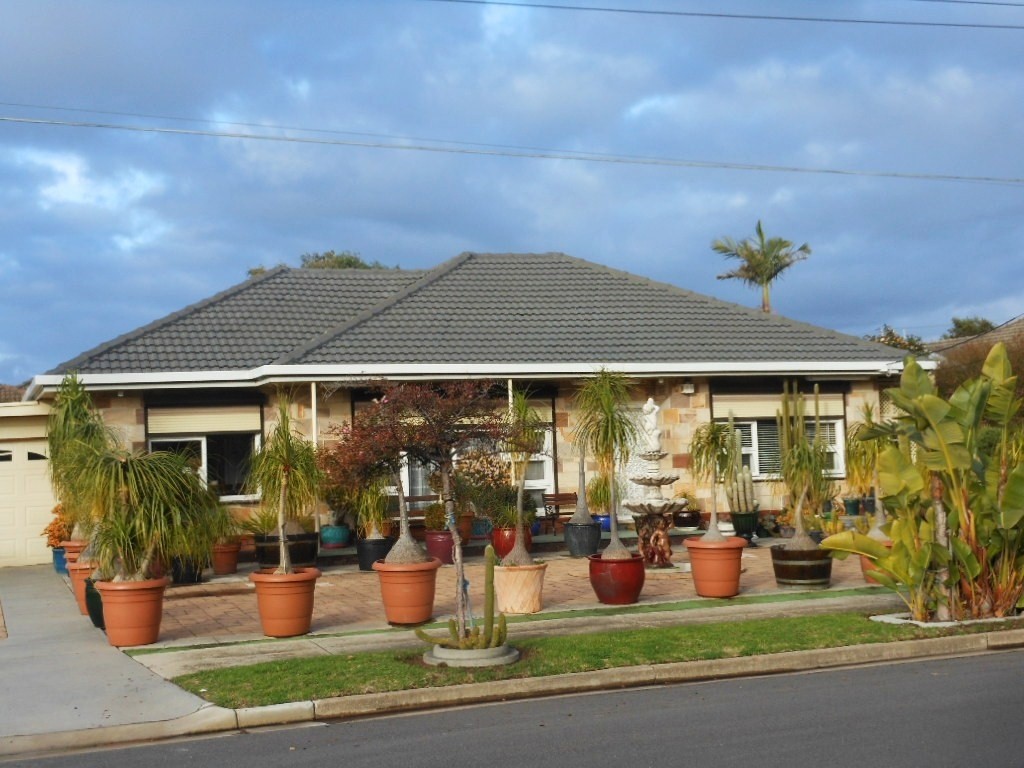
[40,504,70,550]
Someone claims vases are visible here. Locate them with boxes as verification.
[54,550,69,576]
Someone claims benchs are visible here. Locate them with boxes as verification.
[377,494,442,541]
[540,492,580,535]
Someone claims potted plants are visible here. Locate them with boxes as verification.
[49,367,923,648]
